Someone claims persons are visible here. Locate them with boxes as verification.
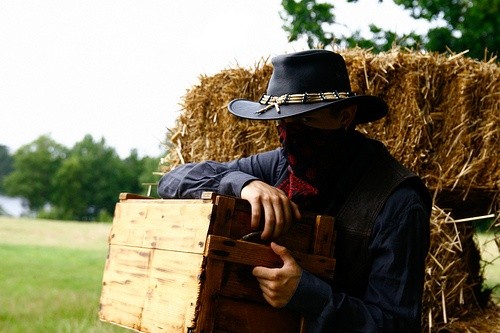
[156,47,433,332]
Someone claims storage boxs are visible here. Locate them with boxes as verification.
[97,189,336,333]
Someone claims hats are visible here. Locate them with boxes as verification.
[227,49,390,127]
